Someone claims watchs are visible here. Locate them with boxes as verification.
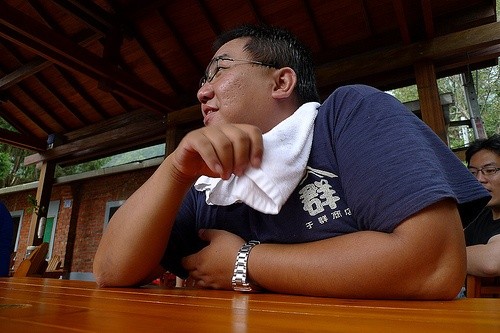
[231,240,259,292]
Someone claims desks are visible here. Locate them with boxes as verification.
[0,273,500,333]
[44,269,64,279]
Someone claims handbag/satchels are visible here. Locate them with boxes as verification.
[159,273,177,288]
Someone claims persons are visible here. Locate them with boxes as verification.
[466,134,500,299]
[93,29,467,300]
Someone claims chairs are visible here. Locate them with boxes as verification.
[11,241,61,278]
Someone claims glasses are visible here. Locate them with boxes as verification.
[469,166,500,176]
[198,57,279,88]
